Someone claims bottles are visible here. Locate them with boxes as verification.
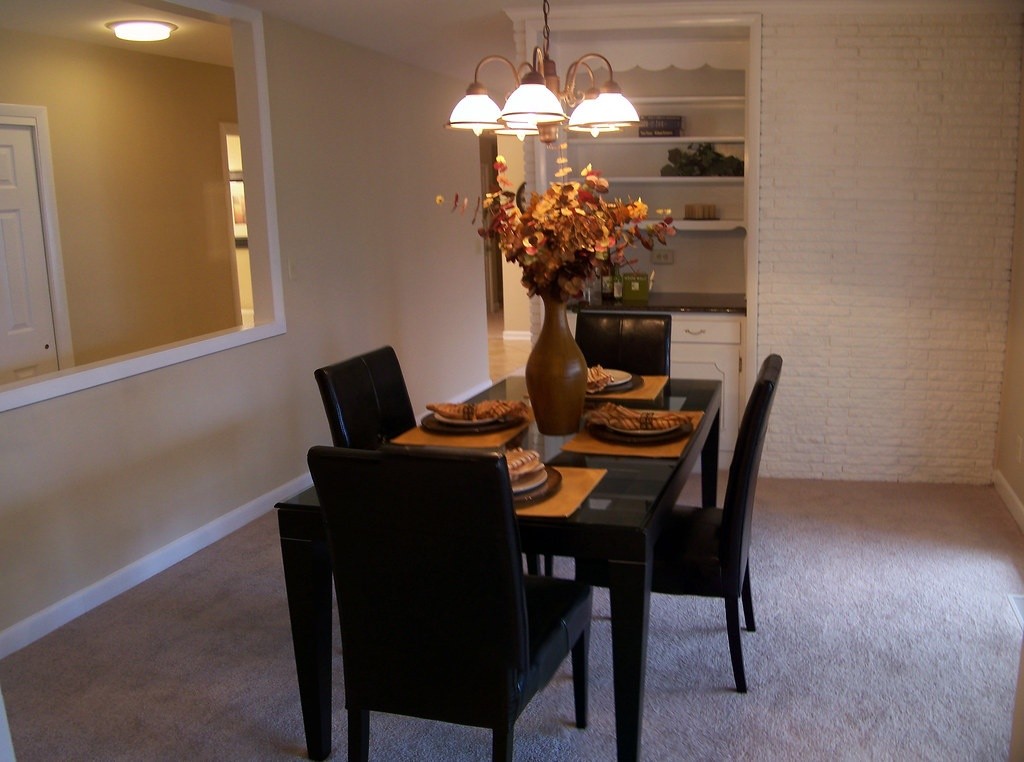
[601,249,614,303]
[614,263,623,302]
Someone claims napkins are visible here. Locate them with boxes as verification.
[490,449,545,480]
[426,398,526,424]
[581,402,694,434]
[584,364,613,394]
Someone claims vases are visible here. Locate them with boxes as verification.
[526,295,589,440]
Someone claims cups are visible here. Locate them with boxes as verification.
[578,289,591,307]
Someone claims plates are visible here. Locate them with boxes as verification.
[607,410,680,434]
[512,469,548,493]
[433,412,497,424]
[601,369,632,385]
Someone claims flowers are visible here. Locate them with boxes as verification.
[435,144,679,305]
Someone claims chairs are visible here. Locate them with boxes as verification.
[314,346,418,445]
[307,446,593,762]
[544,311,672,577]
[574,354,783,693]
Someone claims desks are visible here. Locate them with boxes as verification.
[273,376,722,762]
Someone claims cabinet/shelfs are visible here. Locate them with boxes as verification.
[526,12,762,472]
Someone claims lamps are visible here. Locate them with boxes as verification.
[108,20,178,42]
[444,0,640,146]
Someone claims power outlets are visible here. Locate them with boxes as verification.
[652,249,674,264]
